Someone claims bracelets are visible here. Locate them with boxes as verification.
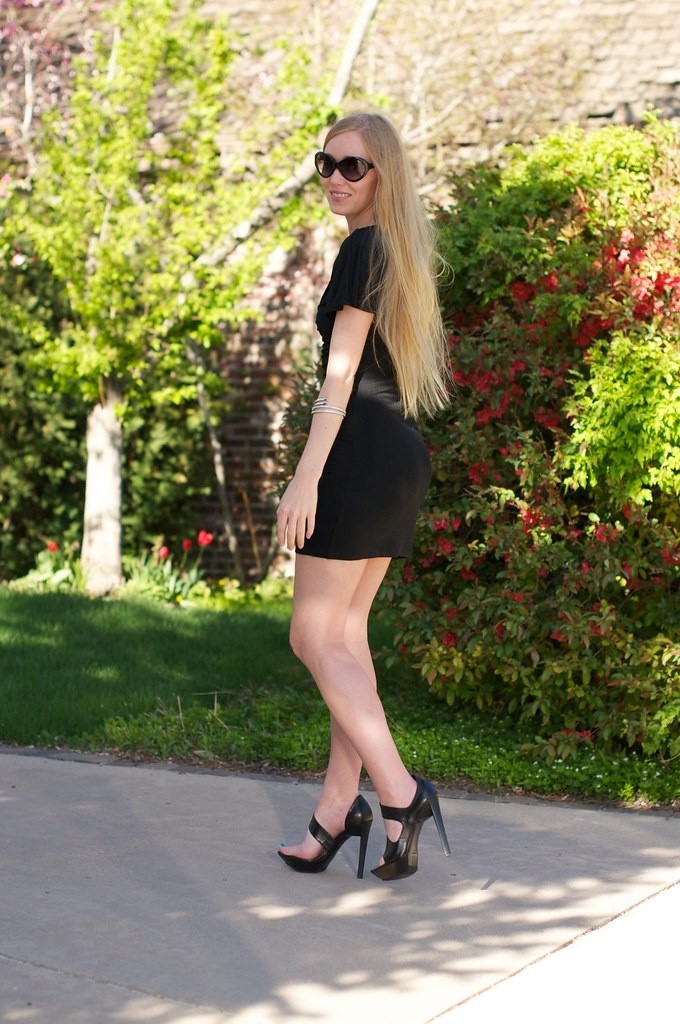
[311,398,346,417]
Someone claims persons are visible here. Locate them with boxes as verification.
[277,114,452,882]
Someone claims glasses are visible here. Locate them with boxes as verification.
[315,152,375,182]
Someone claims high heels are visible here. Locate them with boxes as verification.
[277,794,373,878]
[370,774,451,881]
